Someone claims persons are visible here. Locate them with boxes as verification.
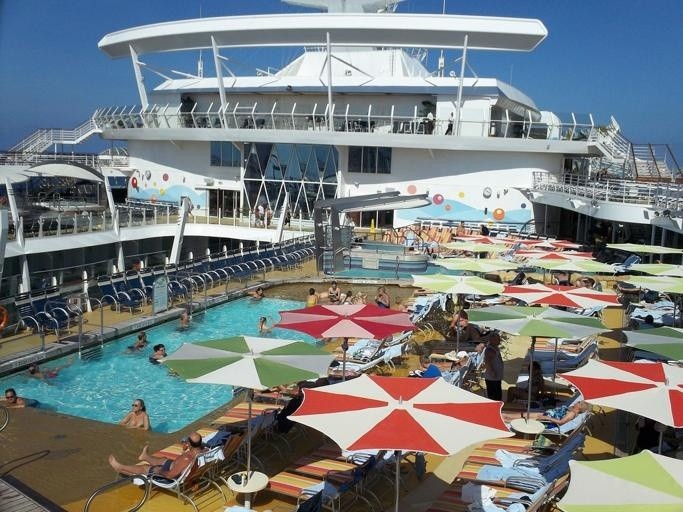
[174,308,192,333]
[241,286,265,306]
[252,201,290,229]
[341,213,351,226]
[383,220,491,252]
[336,289,353,305]
[349,216,355,228]
[426,112,435,134]
[444,111,453,135]
[115,398,150,432]
[149,343,167,365]
[303,288,318,308]
[0,388,38,409]
[121,331,149,355]
[256,316,273,336]
[106,431,205,481]
[328,281,341,305]
[372,286,390,308]
[27,364,62,386]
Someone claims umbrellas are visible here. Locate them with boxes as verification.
[285,372,516,512]
[156,333,336,474]
[271,302,416,383]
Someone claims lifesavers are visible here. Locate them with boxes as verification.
[0,307,8,331]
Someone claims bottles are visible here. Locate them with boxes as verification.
[239,473,247,486]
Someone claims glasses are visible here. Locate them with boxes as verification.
[131,403,141,408]
[4,395,16,400]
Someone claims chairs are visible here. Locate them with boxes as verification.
[385,224,524,260]
[119,384,416,511]
[96,251,260,316]
[14,287,88,353]
[610,255,682,326]
[185,116,429,135]
[431,336,598,511]
[233,232,317,282]
[331,292,502,389]
